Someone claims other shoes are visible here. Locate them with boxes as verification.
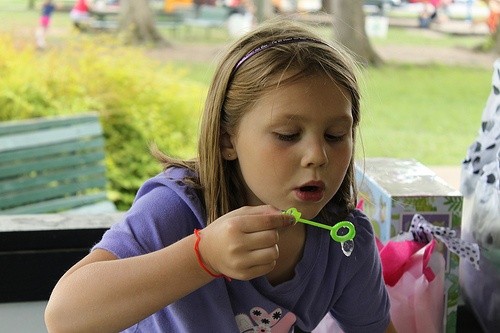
[69,10,94,33]
[35,25,46,50]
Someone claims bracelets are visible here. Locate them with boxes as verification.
[194,228,232,282]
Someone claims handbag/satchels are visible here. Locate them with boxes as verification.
[311,196,445,333]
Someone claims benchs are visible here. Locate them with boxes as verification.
[84,4,232,41]
[0,114,119,213]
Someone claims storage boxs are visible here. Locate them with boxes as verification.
[350,159,463,333]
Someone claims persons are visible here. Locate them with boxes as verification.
[33,0,449,59]
[43,23,401,333]
